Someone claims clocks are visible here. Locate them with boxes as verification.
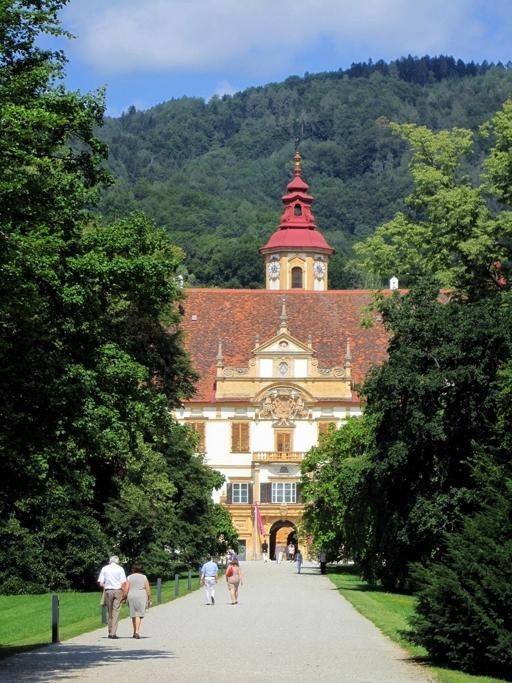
[313,260,327,279]
[267,259,280,280]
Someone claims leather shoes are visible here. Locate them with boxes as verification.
[108,635,117,638]
[134,633,140,639]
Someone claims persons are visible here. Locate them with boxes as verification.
[261,539,268,565]
[287,541,295,563]
[125,564,151,639]
[97,556,126,638]
[319,547,328,574]
[225,559,243,604]
[295,549,302,573]
[200,554,218,605]
[274,540,282,564]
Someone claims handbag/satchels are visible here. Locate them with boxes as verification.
[227,567,233,576]
